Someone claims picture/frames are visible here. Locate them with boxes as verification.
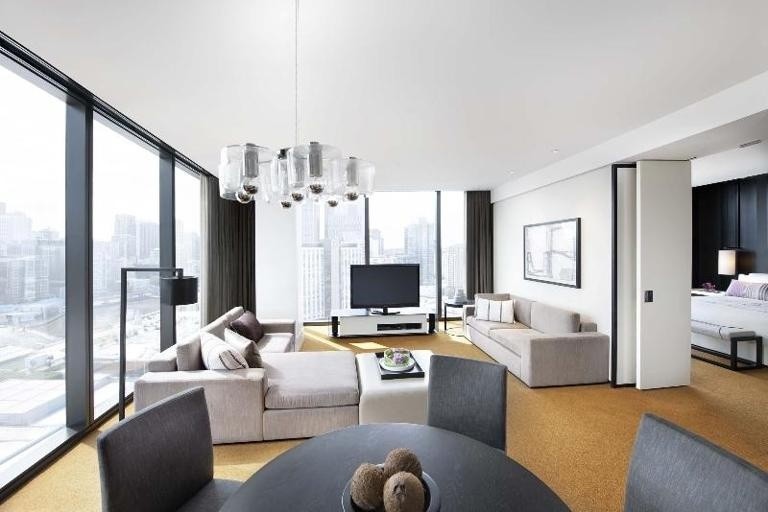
[523,217,584,290]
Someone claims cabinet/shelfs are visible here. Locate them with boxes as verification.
[329,309,436,338]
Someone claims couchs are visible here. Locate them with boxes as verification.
[462,289,608,387]
[133,304,442,441]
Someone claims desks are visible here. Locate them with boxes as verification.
[220,420,573,510]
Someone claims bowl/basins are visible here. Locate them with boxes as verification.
[340,462,442,512]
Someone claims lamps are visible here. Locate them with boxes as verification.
[119,266,198,419]
[220,0,376,209]
[716,245,738,276]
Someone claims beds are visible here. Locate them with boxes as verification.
[690,272,768,366]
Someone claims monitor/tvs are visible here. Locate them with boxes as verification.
[350,263,421,315]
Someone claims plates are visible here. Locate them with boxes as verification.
[380,357,415,371]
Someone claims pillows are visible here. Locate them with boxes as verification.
[725,279,768,301]
[198,310,265,369]
[474,293,514,323]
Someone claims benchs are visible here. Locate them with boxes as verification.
[691,315,762,371]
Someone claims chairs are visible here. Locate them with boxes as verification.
[614,410,767,511]
[428,355,509,450]
[93,385,247,511]
